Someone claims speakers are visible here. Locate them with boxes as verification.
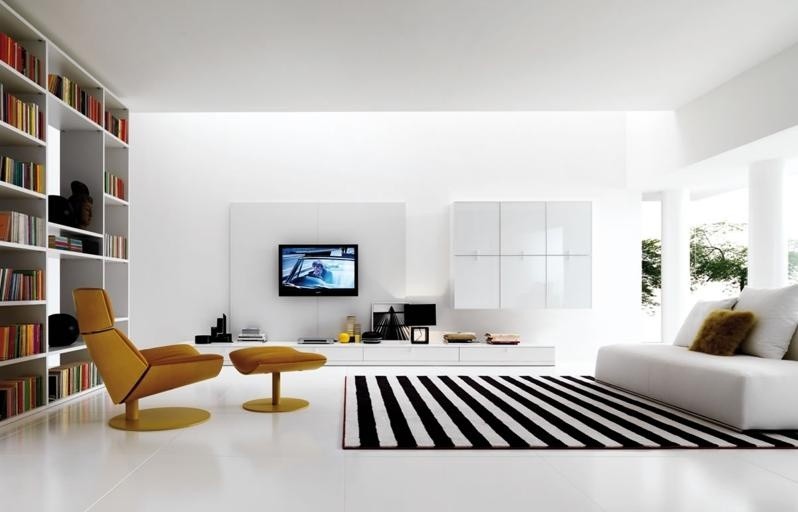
[48,314,79,347]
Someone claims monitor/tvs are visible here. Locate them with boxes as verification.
[279,244,358,296]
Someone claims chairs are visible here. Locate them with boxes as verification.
[72,287,225,433]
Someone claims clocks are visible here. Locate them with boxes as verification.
[411,326,429,343]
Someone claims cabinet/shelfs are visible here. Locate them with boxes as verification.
[0,0,132,439]
[449,198,595,313]
[186,339,559,367]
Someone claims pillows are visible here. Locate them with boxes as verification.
[672,296,739,350]
[689,307,755,356]
[734,284,798,361]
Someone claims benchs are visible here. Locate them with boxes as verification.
[595,286,798,433]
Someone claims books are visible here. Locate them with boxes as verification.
[1,268,42,301]
[49,235,82,251]
[1,33,40,85]
[2,322,43,361]
[105,171,124,199]
[3,92,43,140]
[1,211,44,247]
[2,157,43,193]
[49,361,101,402]
[105,112,127,142]
[1,376,42,420]
[106,234,127,259]
[49,74,101,125]
[237,328,265,341]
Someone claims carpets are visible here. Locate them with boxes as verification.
[340,373,797,451]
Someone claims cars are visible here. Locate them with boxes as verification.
[281,249,356,292]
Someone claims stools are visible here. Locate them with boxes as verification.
[230,345,328,414]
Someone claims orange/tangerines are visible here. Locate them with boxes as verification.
[338,333,350,343]
